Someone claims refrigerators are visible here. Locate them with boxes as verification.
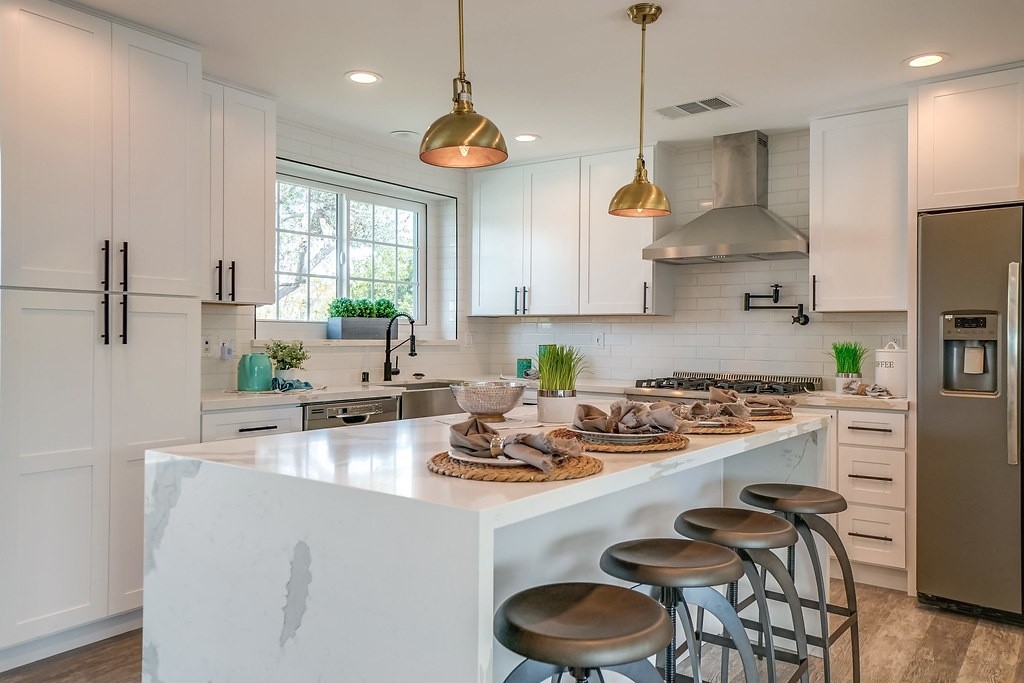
[915,204,1024,624]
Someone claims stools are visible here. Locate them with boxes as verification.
[493,482,860,683]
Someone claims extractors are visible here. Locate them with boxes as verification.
[642,130,809,265]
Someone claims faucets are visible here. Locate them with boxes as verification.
[382,311,418,382]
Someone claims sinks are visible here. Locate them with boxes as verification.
[376,382,467,420]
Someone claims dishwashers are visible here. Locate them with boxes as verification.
[302,398,398,431]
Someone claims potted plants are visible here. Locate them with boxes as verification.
[524,342,596,423]
[823,340,871,393]
[326,297,398,340]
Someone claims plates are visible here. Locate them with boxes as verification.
[686,420,729,426]
[566,424,669,441]
[750,408,781,416]
[447,430,552,465]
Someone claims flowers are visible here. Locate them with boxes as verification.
[265,338,310,373]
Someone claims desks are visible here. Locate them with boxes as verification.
[143,397,830,683]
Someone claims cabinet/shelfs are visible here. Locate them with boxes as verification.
[0,2,304,677]
[523,388,908,593]
[808,65,1024,313]
[466,146,675,317]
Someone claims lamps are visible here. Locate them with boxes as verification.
[419,1,508,169]
[608,2,671,218]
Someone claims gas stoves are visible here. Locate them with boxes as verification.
[625,371,822,405]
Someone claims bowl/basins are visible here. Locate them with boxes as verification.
[449,381,526,422]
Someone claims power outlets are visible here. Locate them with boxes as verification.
[591,331,605,349]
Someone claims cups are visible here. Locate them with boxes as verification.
[517,359,532,377]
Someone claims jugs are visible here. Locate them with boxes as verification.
[238,353,273,390]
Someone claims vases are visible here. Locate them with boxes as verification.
[275,370,296,381]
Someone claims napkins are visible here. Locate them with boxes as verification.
[449,387,799,474]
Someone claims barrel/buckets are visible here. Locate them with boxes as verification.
[874,341,907,397]
